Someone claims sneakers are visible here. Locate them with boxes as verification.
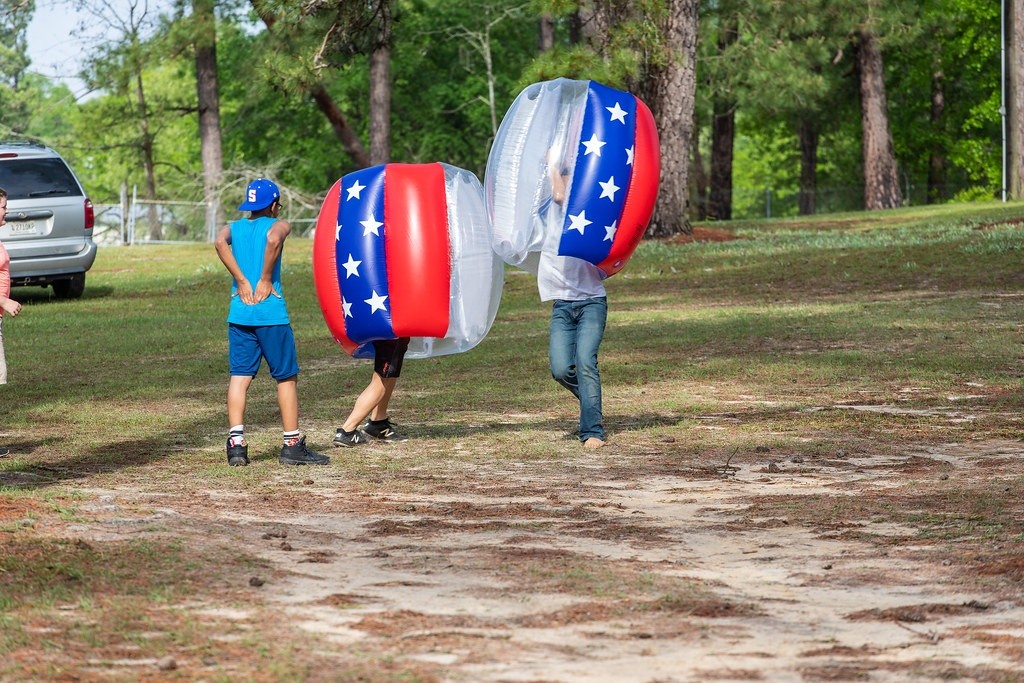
[279,435,329,465]
[226,443,250,466]
[333,428,368,448]
[364,418,409,443]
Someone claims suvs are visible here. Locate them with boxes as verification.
[0,140,97,300]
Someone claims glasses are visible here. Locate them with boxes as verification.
[276,202,283,210]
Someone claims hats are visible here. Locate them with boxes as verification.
[238,179,280,211]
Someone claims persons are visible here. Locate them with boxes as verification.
[213,178,330,469]
[332,337,412,449]
[1,189,24,456]
[535,148,610,451]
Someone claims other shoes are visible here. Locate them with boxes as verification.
[0,448,9,456]
